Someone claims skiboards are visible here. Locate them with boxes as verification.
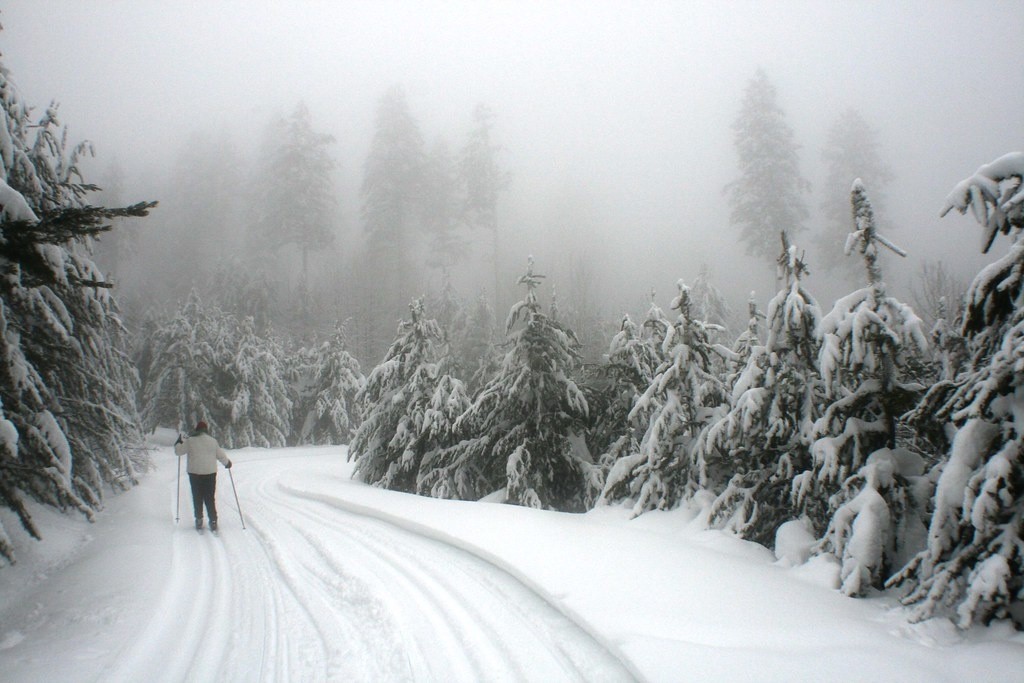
[195,526,220,538]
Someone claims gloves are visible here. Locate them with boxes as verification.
[174,438,183,445]
[225,461,232,468]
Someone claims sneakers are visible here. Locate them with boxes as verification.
[209,517,218,530]
[196,519,203,529]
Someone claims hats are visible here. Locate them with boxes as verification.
[196,422,207,431]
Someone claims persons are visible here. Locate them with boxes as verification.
[173,423,232,531]
